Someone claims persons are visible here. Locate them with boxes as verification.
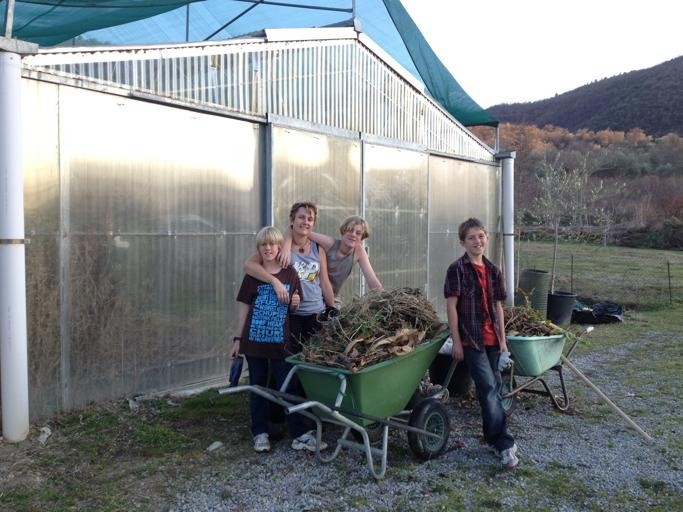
[292,240,310,253]
[444,217,523,466]
[228,225,327,452]
[308,215,382,305]
[280,203,337,415]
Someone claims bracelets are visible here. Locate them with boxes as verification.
[233,336,241,342]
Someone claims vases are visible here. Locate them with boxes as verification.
[517,269,553,323]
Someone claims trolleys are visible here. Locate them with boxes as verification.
[497,325,594,417]
[217,322,457,480]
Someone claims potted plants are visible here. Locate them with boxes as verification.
[523,152,577,329]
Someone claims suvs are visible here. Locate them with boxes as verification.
[75,211,233,303]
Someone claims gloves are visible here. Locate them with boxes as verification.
[498,352,514,373]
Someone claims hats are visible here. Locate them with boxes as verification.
[229,356,243,386]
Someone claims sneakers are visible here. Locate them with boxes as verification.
[487,442,519,469]
[290,430,328,453]
[253,429,271,453]
